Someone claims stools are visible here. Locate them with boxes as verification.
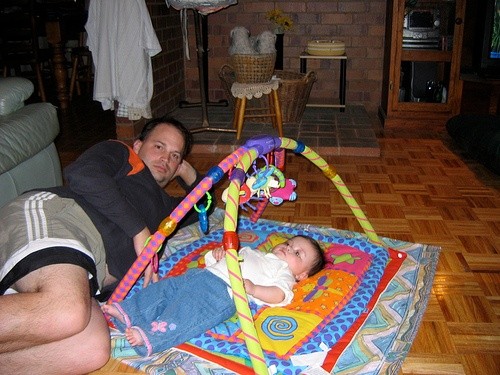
[231,81,283,140]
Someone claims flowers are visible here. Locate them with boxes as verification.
[264,9,294,32]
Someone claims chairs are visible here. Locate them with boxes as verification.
[69,11,91,100]
[0,13,46,102]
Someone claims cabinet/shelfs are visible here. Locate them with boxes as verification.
[300,51,347,112]
[379,0,466,128]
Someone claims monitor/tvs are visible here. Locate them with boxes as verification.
[402,8,441,39]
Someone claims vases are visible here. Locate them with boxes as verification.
[273,25,284,34]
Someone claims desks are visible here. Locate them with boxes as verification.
[38,8,74,112]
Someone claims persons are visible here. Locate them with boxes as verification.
[99,234,328,347]
[0,116,217,375]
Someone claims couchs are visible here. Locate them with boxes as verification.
[0,77,62,207]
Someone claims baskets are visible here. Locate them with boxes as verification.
[217,64,317,124]
[232,51,276,84]
